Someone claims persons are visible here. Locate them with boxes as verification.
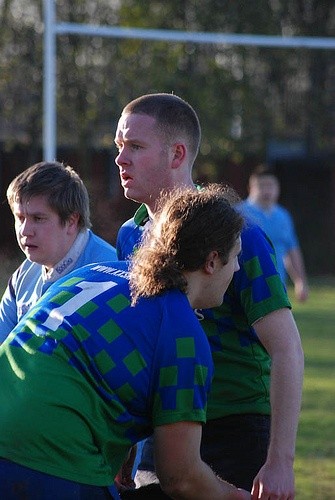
[0,161,116,342]
[0,185,260,500]
[231,163,309,304]
[113,92,304,499]
